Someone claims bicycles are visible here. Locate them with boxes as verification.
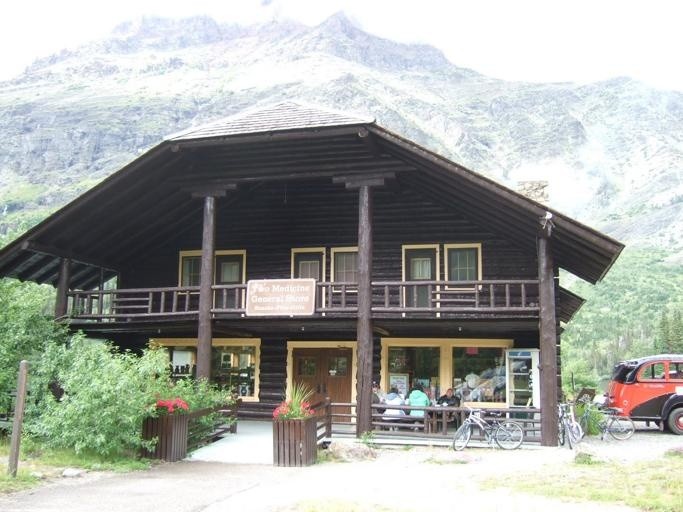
[453,402,524,452]
[557,394,636,450]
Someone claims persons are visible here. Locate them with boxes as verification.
[402,384,428,431]
[378,387,404,432]
[436,387,460,418]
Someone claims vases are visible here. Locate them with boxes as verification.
[272,415,316,468]
[140,411,188,461]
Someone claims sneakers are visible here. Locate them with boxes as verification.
[409,420,420,432]
[387,421,398,433]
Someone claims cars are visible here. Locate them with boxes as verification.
[607,354,683,435]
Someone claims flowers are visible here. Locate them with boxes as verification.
[148,394,187,414]
[268,379,314,418]
